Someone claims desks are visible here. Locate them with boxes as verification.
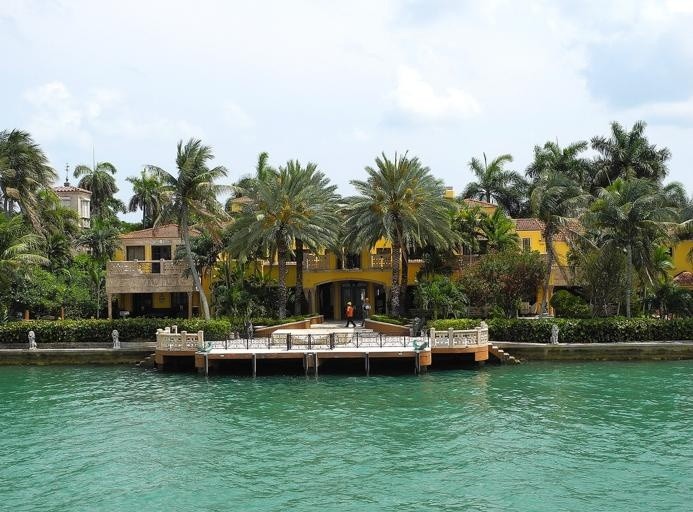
[272,328,356,344]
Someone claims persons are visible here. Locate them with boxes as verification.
[361,297,371,326]
[344,300,355,329]
